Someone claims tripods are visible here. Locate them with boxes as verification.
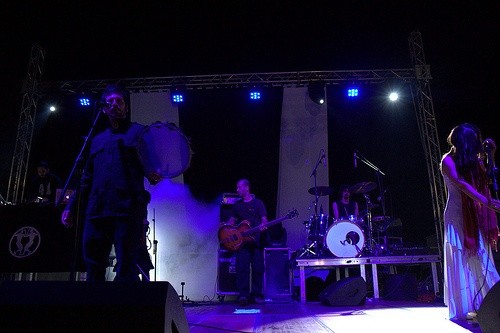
[296,162,391,259]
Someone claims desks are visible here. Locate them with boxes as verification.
[295,255,443,303]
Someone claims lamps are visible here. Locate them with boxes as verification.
[79,93,91,106]
[249,88,261,100]
[172,90,184,103]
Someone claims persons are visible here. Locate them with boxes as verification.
[441,124,500,324]
[58,88,158,282]
[219,179,268,305]
[333,186,359,222]
[27,161,61,201]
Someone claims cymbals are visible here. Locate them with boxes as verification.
[350,182,378,193]
[308,186,334,196]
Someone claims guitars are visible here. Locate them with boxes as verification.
[218,206,301,250]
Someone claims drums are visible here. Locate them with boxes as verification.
[308,215,334,241]
[137,123,191,179]
[324,219,365,258]
[341,214,364,228]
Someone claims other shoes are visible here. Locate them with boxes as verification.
[249,295,266,304]
[238,297,248,306]
[466,315,473,320]
[468,311,478,317]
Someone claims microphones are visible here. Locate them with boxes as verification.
[353,148,358,168]
[94,101,111,108]
[322,150,327,167]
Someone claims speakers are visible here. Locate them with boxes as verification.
[216,250,252,302]
[382,271,418,302]
[0,281,188,333]
[265,247,292,297]
[318,277,369,308]
[477,279,500,333]
